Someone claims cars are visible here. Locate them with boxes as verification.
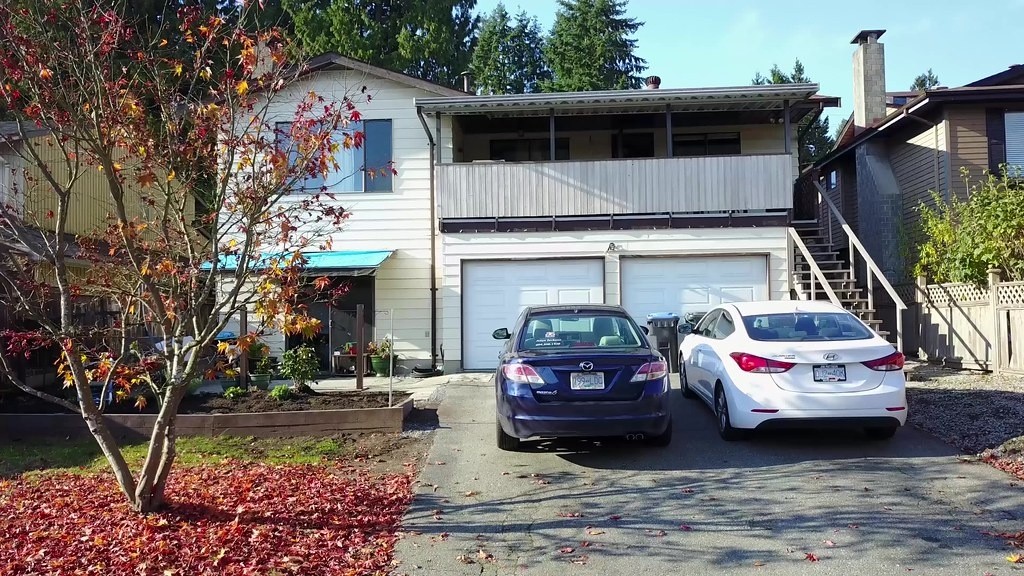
[674,300,910,443]
[492,303,674,450]
[55,359,168,411]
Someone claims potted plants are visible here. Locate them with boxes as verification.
[368,336,399,377]
[250,359,270,390]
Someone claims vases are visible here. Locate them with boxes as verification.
[220,379,240,391]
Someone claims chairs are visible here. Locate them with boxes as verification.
[794,317,816,336]
[592,318,615,345]
[599,336,621,346]
[819,327,841,338]
[524,320,563,348]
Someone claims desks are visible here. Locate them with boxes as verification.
[332,353,371,377]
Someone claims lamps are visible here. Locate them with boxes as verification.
[768,113,775,124]
[778,117,784,124]
